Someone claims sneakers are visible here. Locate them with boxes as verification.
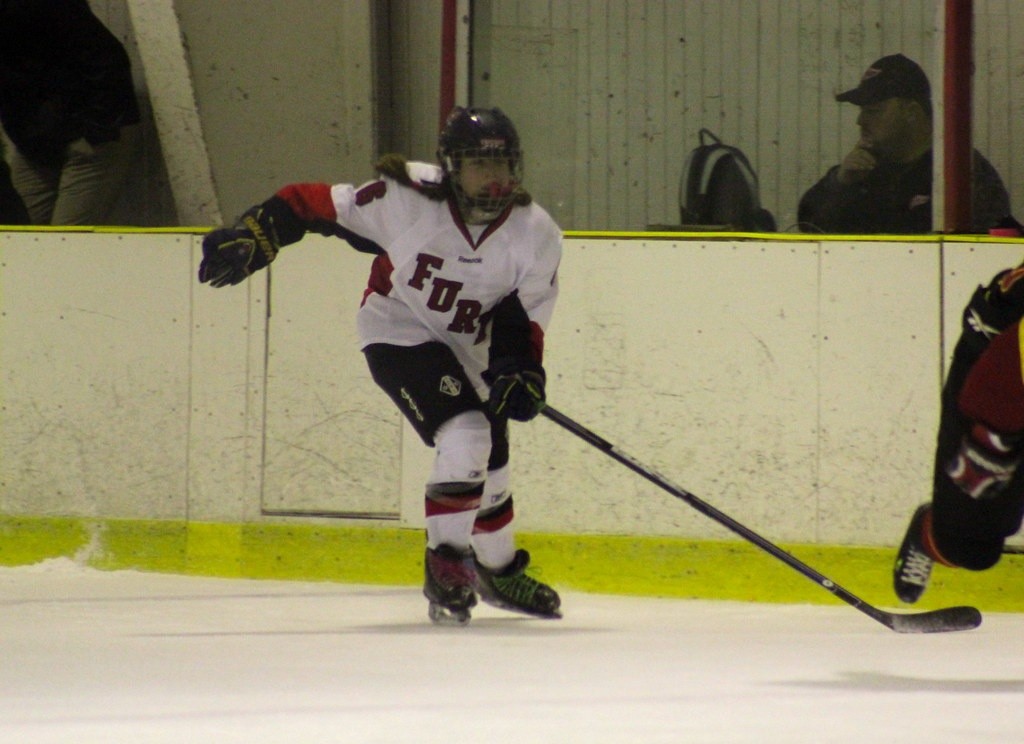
[893,505,932,606]
[422,543,479,625]
[474,548,562,619]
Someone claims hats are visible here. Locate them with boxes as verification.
[834,54,929,105]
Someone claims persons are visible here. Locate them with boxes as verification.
[0,0,138,228]
[891,263,1024,603]
[199,106,563,630]
[797,52,1011,234]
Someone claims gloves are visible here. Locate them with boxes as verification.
[481,355,547,420]
[198,205,281,289]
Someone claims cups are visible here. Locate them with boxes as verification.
[988,218,1019,238]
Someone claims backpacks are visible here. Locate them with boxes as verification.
[680,130,774,232]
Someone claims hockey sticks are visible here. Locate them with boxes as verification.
[539,401,986,638]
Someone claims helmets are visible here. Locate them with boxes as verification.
[440,105,521,214]
